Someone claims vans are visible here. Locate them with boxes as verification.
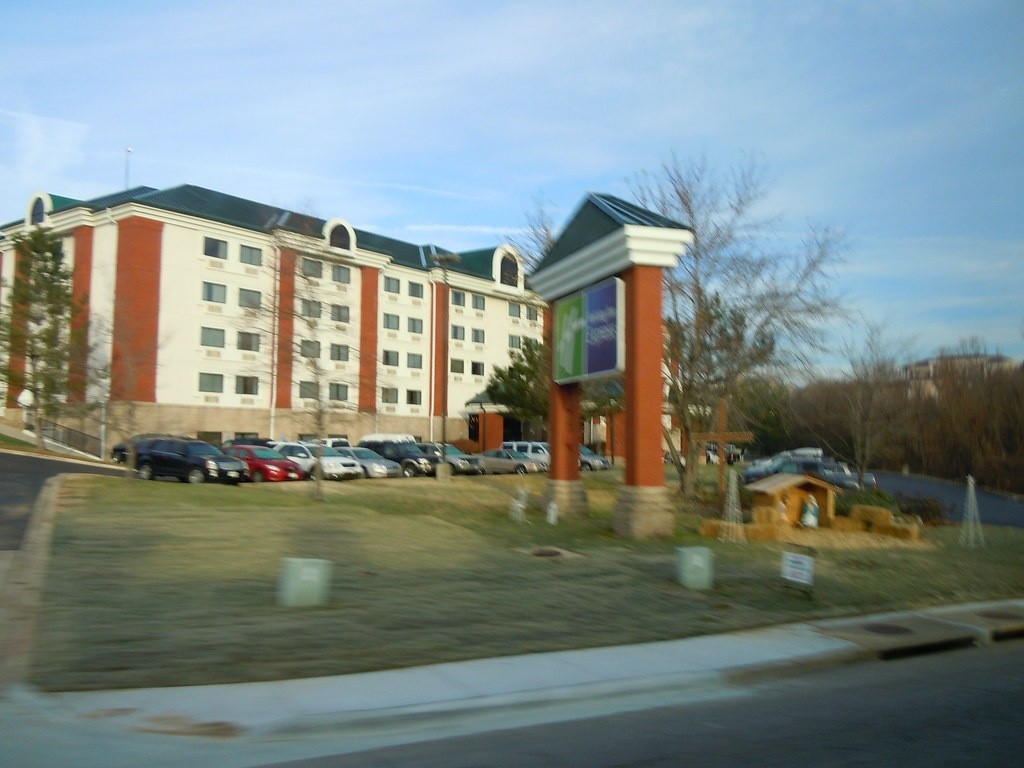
[500,442,582,469]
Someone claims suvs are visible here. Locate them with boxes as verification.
[359,442,442,479]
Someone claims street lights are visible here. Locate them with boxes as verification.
[432,254,462,445]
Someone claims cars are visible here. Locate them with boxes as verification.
[706,450,722,464]
[754,449,852,474]
[137,437,249,486]
[111,433,191,465]
[213,438,274,451]
[219,446,305,483]
[729,445,741,459]
[329,446,404,478]
[468,447,545,476]
[580,446,610,471]
[266,439,362,483]
[437,443,481,475]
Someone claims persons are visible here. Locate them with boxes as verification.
[707,452,710,464]
[727,451,736,465]
[802,495,819,528]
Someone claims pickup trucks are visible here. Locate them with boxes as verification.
[299,439,348,449]
[742,457,877,493]
